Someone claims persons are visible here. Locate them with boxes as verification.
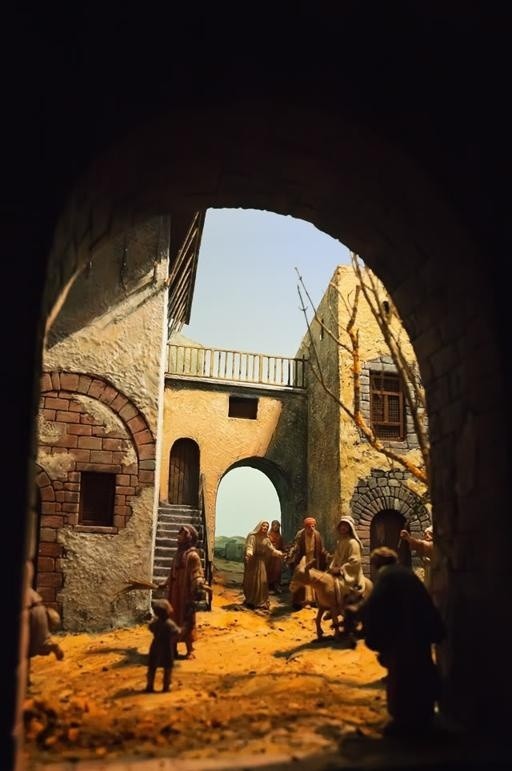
[158,525,213,660]
[362,547,443,737]
[29,590,64,660]
[242,515,363,633]
[145,598,186,693]
[400,525,433,554]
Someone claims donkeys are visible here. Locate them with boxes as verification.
[289,555,374,644]
